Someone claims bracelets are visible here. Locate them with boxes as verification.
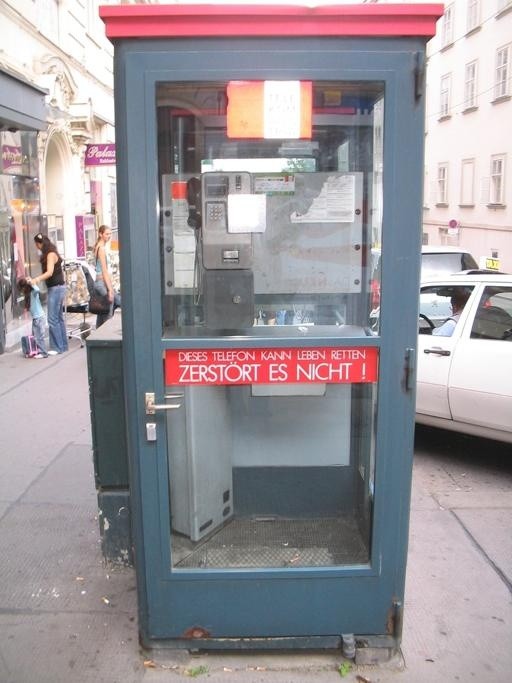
[35,275,41,284]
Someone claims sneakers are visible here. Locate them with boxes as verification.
[33,350,58,359]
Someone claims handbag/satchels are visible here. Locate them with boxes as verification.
[90,296,110,314]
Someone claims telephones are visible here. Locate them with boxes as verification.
[186,171,253,270]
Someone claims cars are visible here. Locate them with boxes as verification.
[366,267,512,453]
[367,245,478,309]
[1,255,14,302]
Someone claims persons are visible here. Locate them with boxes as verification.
[430,291,471,337]
[15,275,50,360]
[25,231,70,356]
[92,224,115,329]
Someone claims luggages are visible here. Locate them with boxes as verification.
[22,309,38,357]
